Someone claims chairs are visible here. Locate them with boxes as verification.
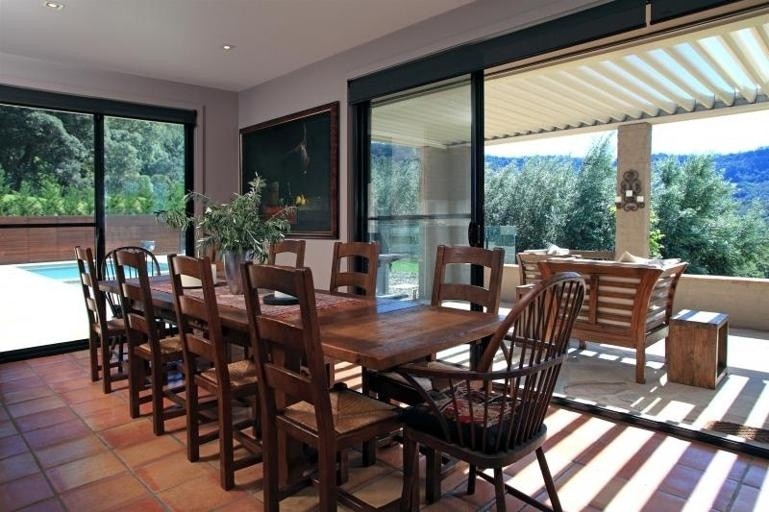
[390,270,586,512]
[166,251,258,493]
[325,237,381,296]
[73,247,134,396]
[112,248,184,437]
[238,258,416,511]
[264,237,309,269]
[427,244,507,314]
[98,244,173,378]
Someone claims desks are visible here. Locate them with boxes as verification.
[96,271,512,496]
[515,247,689,383]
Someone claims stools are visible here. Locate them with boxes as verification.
[666,308,729,390]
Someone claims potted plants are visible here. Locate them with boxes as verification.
[152,180,299,296]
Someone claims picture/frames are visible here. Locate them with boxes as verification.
[238,100,340,240]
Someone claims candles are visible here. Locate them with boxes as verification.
[614,190,644,204]
